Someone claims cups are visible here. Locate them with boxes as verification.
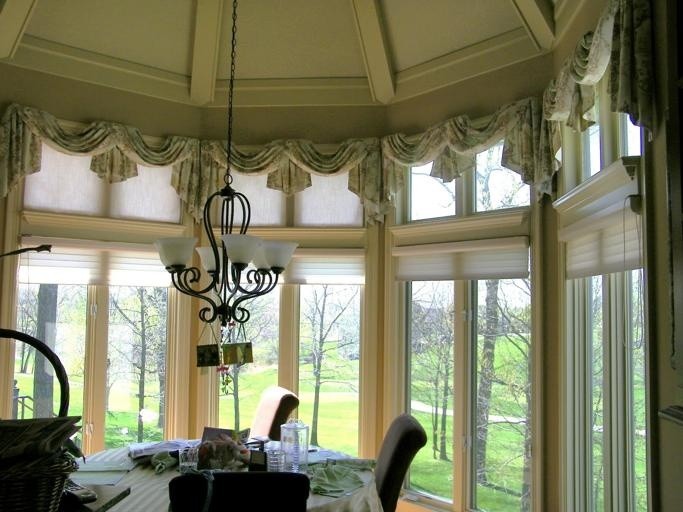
[265,449,287,473]
[177,447,199,474]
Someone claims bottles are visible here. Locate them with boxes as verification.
[280,418,311,476]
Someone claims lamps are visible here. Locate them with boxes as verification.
[151,3,303,370]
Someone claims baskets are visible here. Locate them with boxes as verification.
[1,329,78,511]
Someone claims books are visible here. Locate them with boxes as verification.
[78,482,132,512]
